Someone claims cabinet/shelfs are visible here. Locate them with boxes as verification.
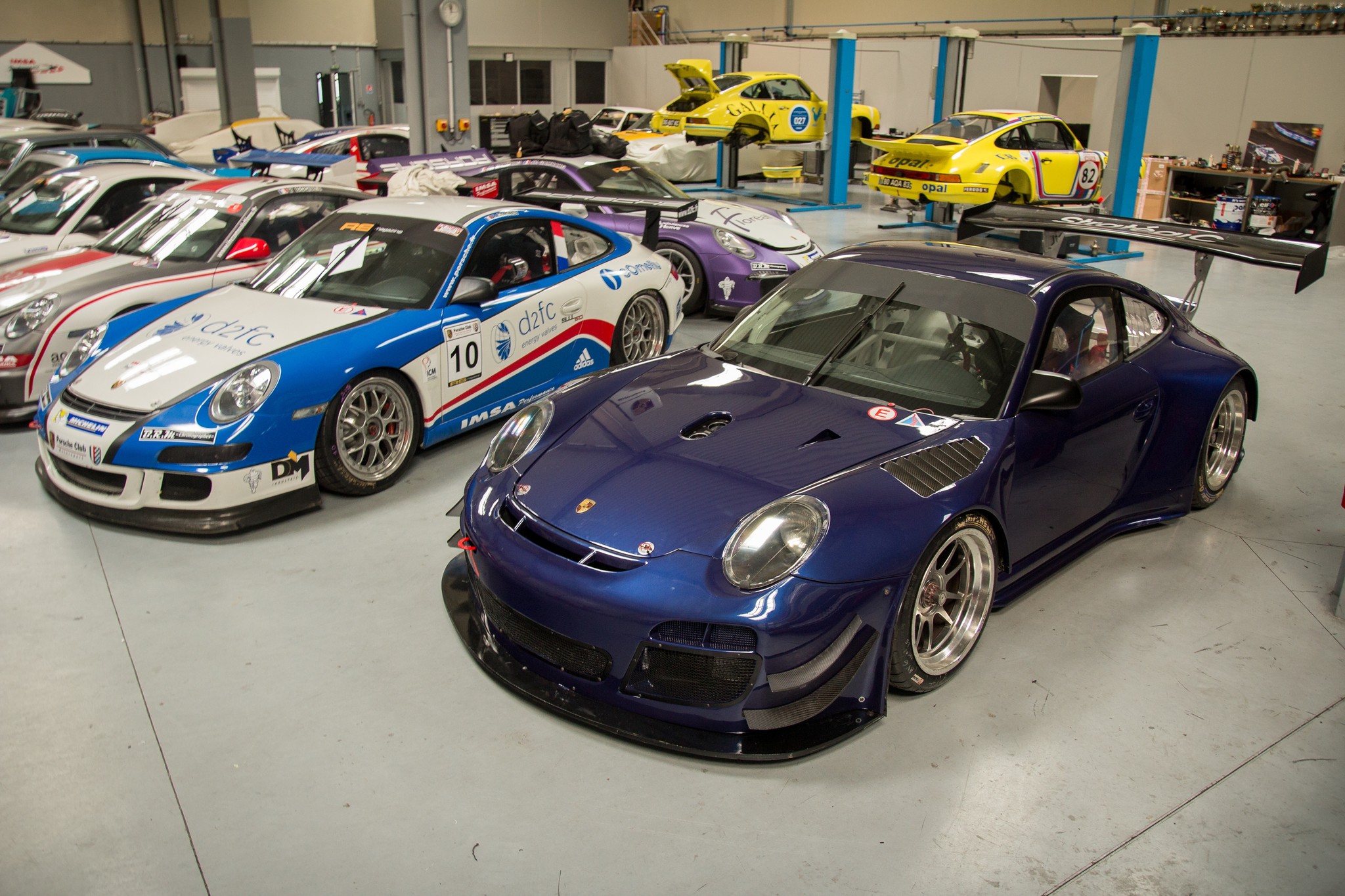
[1160,164,1345,249]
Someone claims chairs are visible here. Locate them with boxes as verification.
[962,123,984,140]
[1043,305,1095,377]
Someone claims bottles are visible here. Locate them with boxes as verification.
[1207,155,1214,168]
[1221,145,1242,171]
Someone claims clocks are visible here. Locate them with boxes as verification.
[438,0,465,28]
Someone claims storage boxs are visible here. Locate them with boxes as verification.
[1133,155,1197,222]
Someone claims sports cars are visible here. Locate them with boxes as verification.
[650,57,883,147]
[861,109,1146,207]
[446,242,1260,760]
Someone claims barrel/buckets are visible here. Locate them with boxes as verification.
[1212,193,1247,232]
[1247,196,1281,229]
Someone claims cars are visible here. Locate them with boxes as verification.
[366,147,836,320]
[0,161,314,295]
[0,123,180,162]
[1,149,250,217]
[264,122,410,190]
[36,192,688,535]
[579,106,658,142]
[609,112,668,143]
[0,177,405,424]
